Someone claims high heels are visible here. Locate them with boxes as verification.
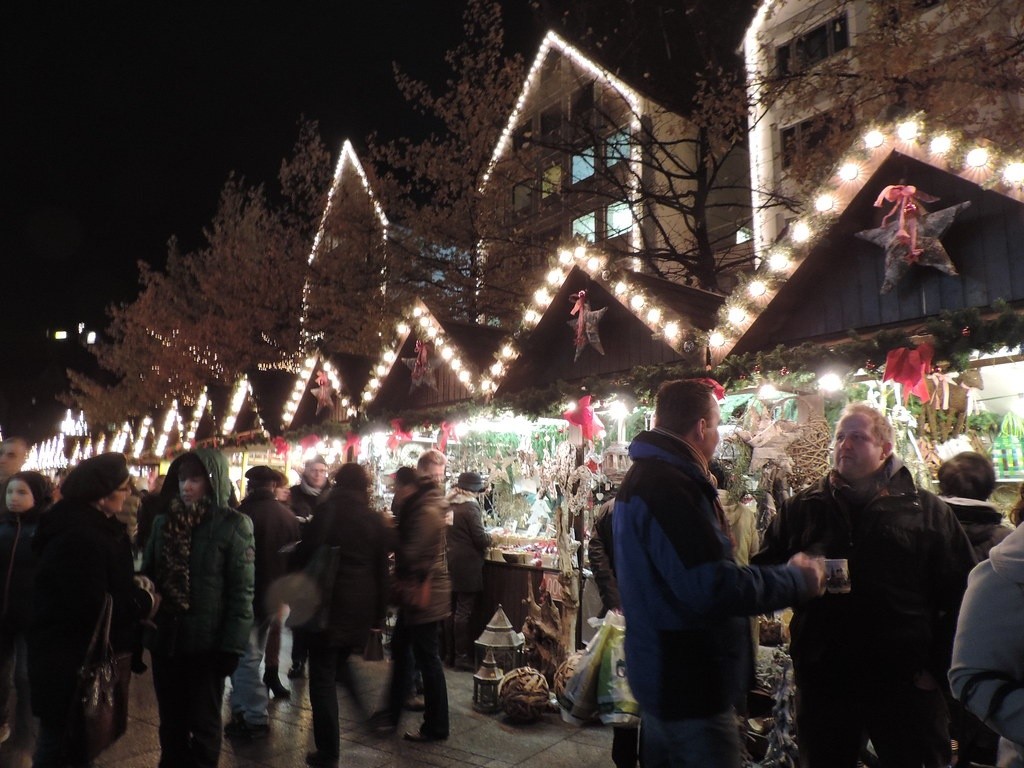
[262,671,290,697]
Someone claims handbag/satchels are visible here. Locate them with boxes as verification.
[68,593,128,760]
[390,577,431,608]
[562,609,641,727]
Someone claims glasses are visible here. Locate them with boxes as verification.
[111,483,131,491]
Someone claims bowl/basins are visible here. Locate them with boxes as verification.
[501,554,534,563]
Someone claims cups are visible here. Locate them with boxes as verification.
[821,559,851,593]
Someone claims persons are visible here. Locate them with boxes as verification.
[750,401,981,768]
[136,447,254,768]
[587,496,646,767]
[932,451,1016,768]
[699,456,761,661]
[611,378,827,767]
[227,451,502,768]
[0,437,166,768]
[947,483,1024,758]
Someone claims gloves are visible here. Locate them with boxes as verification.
[131,642,148,674]
[217,651,240,679]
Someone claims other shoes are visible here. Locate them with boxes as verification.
[305,751,338,768]
[0,724,10,743]
[224,716,270,738]
[404,729,443,740]
[454,655,476,671]
[287,661,305,678]
[406,694,425,712]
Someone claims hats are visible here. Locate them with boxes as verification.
[452,473,485,491]
[5,472,46,508]
[246,467,277,483]
[59,452,130,504]
